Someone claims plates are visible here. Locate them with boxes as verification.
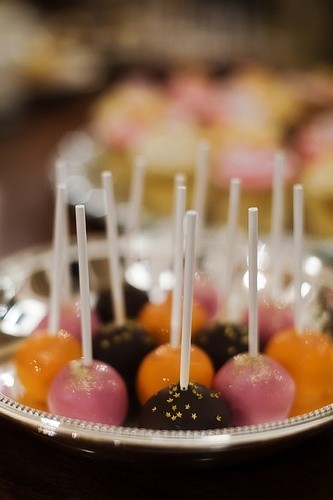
[54,134,332,257]
[0,233,333,455]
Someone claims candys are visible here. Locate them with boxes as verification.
[8,270,333,431]
